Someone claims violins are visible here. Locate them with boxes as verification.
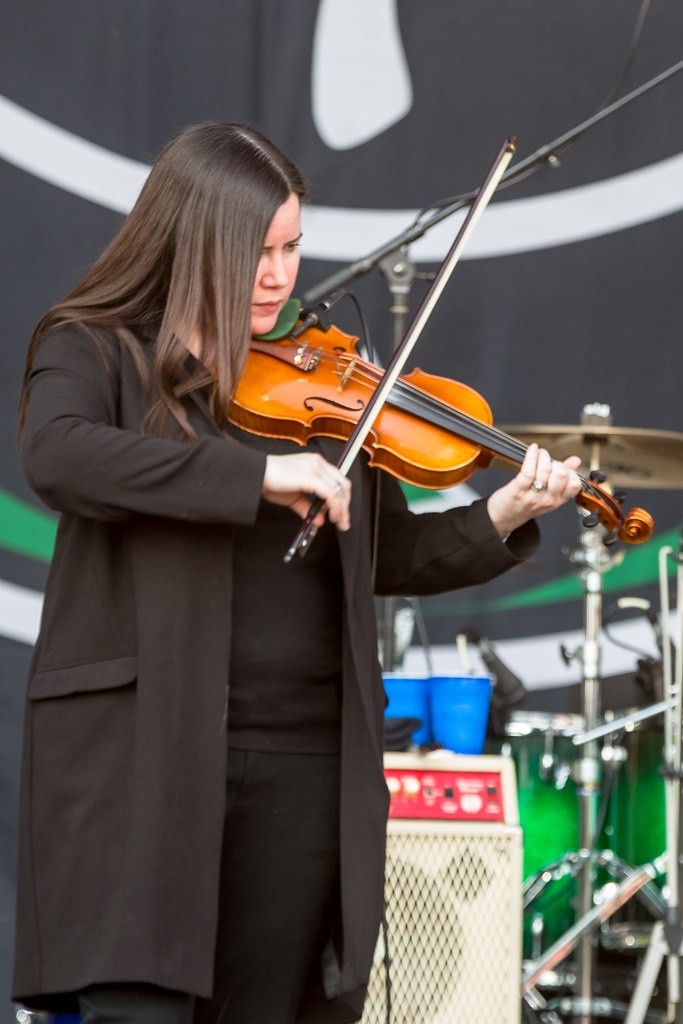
[215,305,657,547]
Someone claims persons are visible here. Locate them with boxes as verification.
[12,121,583,1024]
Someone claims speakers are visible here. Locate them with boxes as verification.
[348,751,522,1024]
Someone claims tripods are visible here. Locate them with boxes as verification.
[518,443,675,1024]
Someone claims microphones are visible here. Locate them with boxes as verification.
[468,628,525,707]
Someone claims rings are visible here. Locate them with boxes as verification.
[530,482,546,492]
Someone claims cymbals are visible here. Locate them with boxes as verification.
[482,420,683,493]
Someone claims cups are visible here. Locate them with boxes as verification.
[426,676,494,756]
[384,671,431,752]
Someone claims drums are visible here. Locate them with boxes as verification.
[601,707,683,955]
[487,710,607,991]
